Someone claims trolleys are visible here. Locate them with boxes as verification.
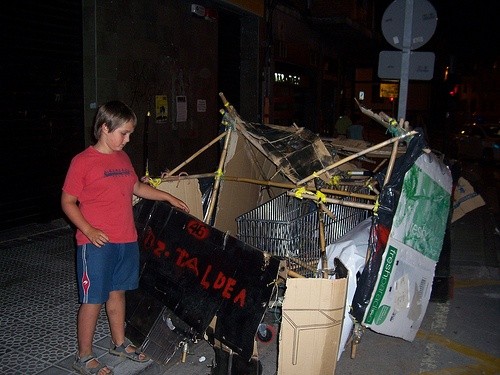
[234,170,379,342]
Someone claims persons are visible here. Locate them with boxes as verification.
[61,98,189,375]
[335,107,366,142]
[466,108,486,145]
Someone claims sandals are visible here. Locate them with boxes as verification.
[72,351,114,375]
[109,340,150,362]
